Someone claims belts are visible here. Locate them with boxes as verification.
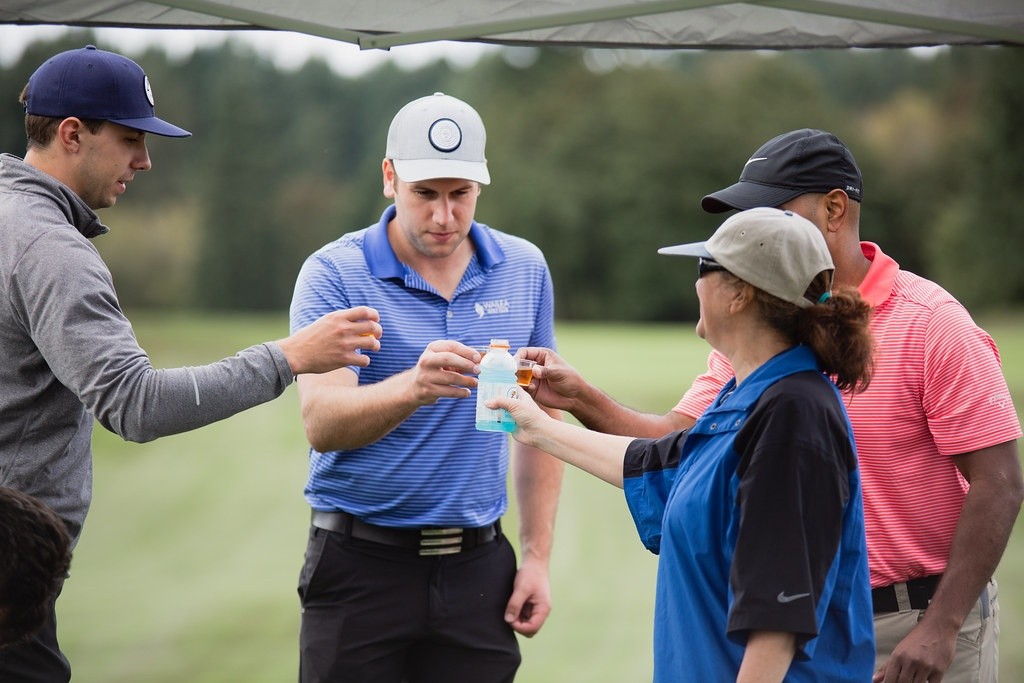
[871,574,944,613]
[312,509,497,555]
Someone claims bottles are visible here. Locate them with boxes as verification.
[475,339,517,432]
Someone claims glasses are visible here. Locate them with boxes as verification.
[697,257,726,278]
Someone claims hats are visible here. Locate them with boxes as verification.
[702,127,863,210]
[658,207,835,307]
[23,45,192,139]
[386,93,491,185]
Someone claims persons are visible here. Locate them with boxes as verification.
[0,44,383,682]
[513,128,1024,683]
[484,206,876,683]
[288,91,563,683]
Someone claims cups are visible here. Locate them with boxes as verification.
[355,319,374,337]
[516,359,537,386]
[468,346,489,365]
